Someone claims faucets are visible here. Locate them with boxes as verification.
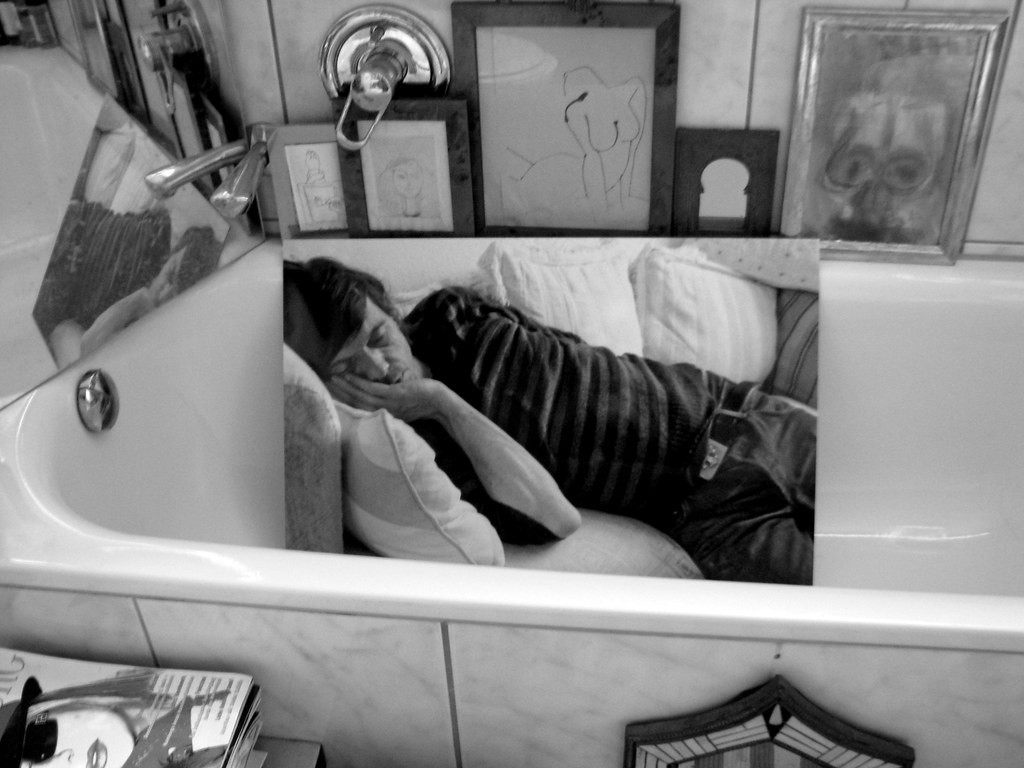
[144,136,247,198]
[207,121,273,218]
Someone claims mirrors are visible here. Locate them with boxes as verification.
[281,236,821,588]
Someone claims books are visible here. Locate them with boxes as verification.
[0,649,266,767]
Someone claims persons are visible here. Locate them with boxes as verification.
[285,255,814,586]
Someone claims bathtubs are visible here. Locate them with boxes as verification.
[2,235,1024,766]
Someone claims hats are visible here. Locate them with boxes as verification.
[0,676,41,768]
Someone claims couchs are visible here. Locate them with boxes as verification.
[282,347,704,579]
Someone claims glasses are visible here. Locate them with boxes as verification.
[22,710,57,768]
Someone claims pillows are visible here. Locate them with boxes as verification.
[626,243,779,390]
[335,401,504,567]
[473,243,640,358]
[768,290,818,404]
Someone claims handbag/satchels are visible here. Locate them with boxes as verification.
[32,91,172,338]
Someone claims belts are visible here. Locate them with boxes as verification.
[699,381,762,480]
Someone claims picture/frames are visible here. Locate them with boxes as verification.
[451,0,681,239]
[778,9,1011,266]
[267,126,350,243]
[330,95,476,239]
[674,127,781,237]
